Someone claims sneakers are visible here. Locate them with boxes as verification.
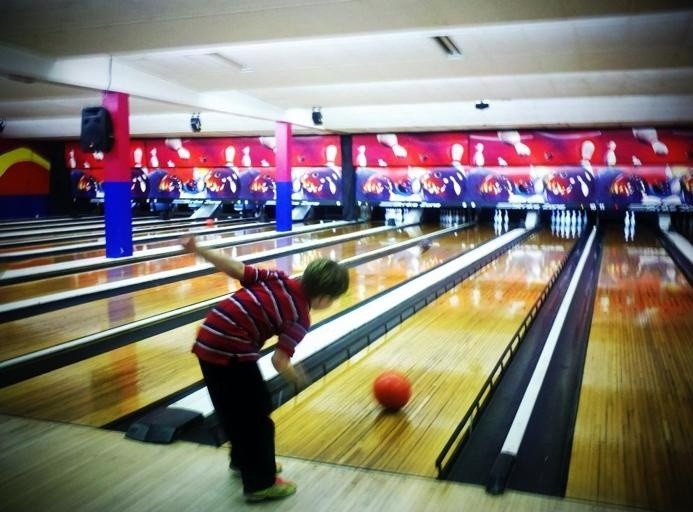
[242,475,299,502]
[229,459,283,478]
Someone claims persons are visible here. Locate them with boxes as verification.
[180,236,350,505]
